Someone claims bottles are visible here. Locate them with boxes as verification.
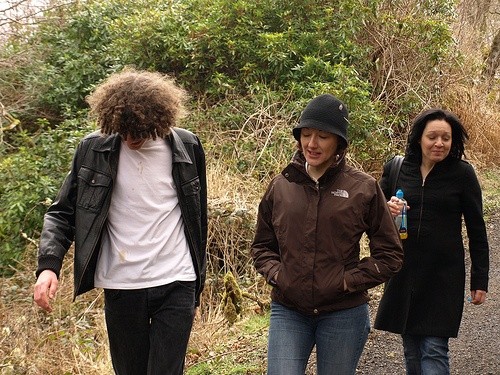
[392,189,408,240]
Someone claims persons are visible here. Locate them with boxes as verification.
[373,109,490,375]
[33,66,208,375]
[249,94,404,375]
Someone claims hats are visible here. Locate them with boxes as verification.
[292,94,348,150]
